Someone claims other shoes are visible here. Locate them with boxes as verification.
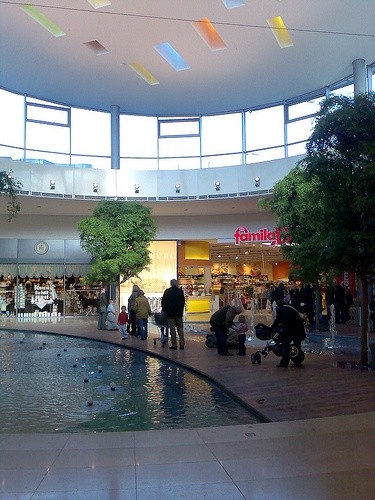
[169,346,177,350]
[180,346,184,350]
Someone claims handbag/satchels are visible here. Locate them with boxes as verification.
[298,313,307,322]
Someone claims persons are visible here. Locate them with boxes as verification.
[162,279,185,350]
[106,300,119,331]
[126,285,140,335]
[117,305,129,340]
[210,306,242,356]
[97,287,109,330]
[275,299,306,368]
[235,315,248,356]
[253,280,375,334]
[134,290,152,340]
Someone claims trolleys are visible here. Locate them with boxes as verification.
[150,311,171,347]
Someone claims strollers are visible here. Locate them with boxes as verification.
[250,322,299,369]
